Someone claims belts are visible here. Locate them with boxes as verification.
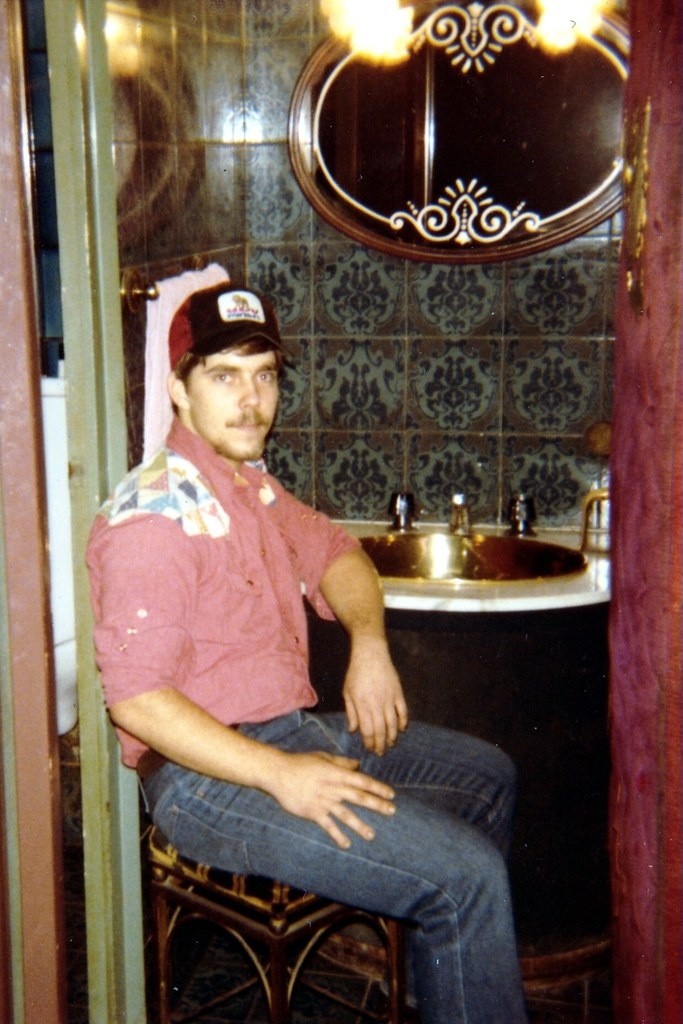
[136,723,240,778]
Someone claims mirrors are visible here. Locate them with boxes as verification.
[288,0,632,265]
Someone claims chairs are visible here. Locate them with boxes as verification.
[131,766,406,1024]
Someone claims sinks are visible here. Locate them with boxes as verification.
[333,523,589,586]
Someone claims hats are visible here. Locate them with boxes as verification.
[167,281,296,370]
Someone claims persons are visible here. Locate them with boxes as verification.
[85,281,532,1024]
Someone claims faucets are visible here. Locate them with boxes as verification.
[450,494,470,536]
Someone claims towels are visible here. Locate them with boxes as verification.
[142,262,229,462]
